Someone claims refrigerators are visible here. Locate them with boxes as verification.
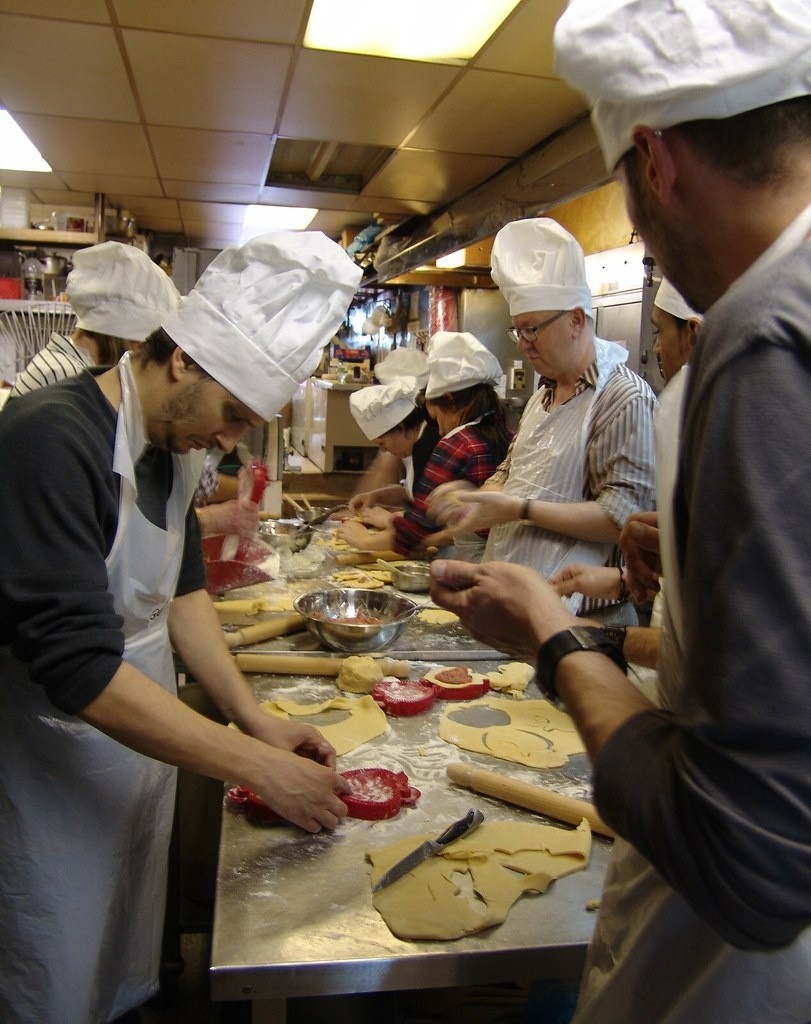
[584,241,666,398]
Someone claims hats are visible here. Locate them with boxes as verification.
[553,0,811,176]
[161,231,364,423]
[654,277,705,322]
[349,376,419,440]
[66,241,183,342]
[490,217,594,319]
[425,331,503,400]
[374,347,428,389]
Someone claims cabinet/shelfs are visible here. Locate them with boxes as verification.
[0,225,96,314]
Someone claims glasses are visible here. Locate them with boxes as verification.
[505,311,570,343]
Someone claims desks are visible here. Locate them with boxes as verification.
[208,658,613,1024]
[209,518,511,661]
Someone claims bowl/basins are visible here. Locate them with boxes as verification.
[294,506,331,525]
[255,522,315,554]
[391,565,433,592]
[294,589,418,652]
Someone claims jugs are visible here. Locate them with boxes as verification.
[38,252,73,274]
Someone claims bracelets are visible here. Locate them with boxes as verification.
[600,622,629,645]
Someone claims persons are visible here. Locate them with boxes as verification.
[425,214,658,629]
[10,242,511,567]
[652,271,703,379]
[0,230,366,1024]
[425,0,811,1024]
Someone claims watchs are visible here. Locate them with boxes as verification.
[519,499,536,526]
[533,626,629,714]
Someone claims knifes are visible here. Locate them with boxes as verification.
[371,808,485,894]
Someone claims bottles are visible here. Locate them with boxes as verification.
[21,251,45,301]
